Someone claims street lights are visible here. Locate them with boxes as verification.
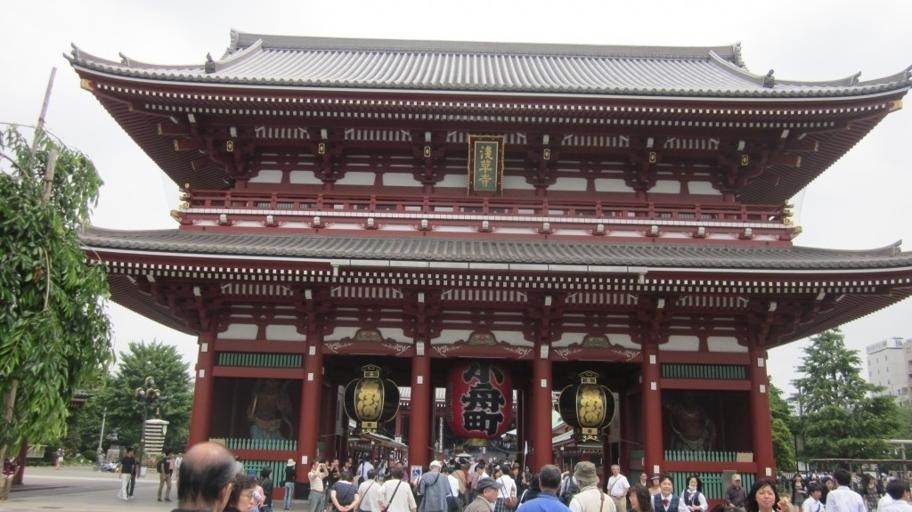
[135,373,161,479]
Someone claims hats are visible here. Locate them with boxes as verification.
[573,461,598,483]
[731,474,741,481]
[287,459,296,467]
[476,477,503,491]
